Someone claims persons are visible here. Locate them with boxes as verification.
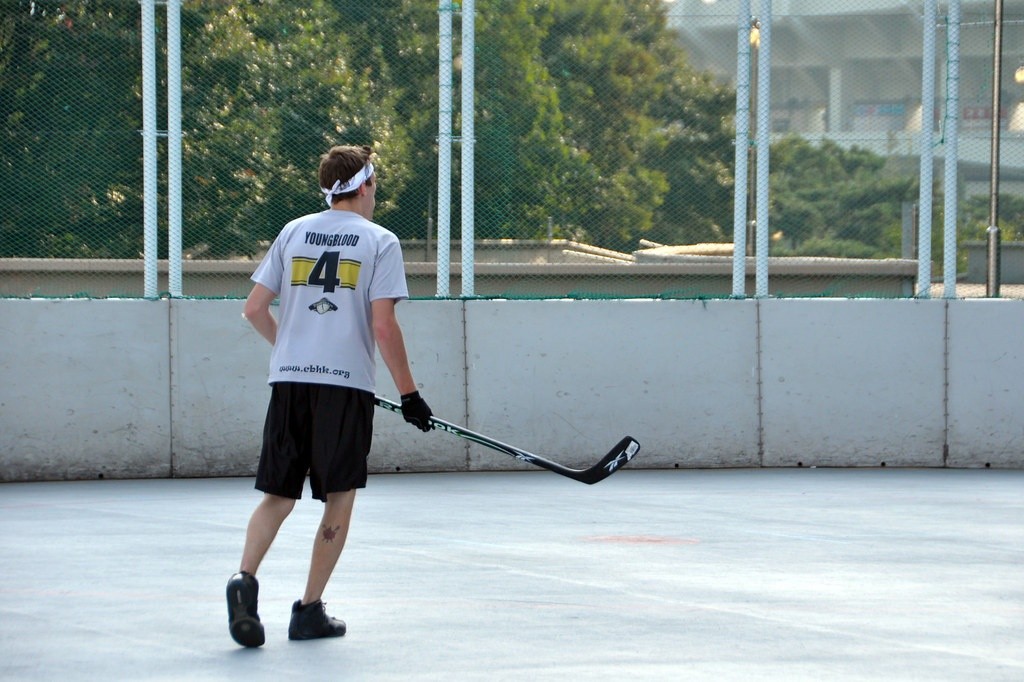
[224,144,435,649]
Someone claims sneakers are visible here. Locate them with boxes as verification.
[289,598,346,641]
[226,571,265,647]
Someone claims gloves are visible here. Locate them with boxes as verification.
[400,390,435,434]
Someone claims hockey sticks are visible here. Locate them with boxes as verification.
[376,394,639,484]
